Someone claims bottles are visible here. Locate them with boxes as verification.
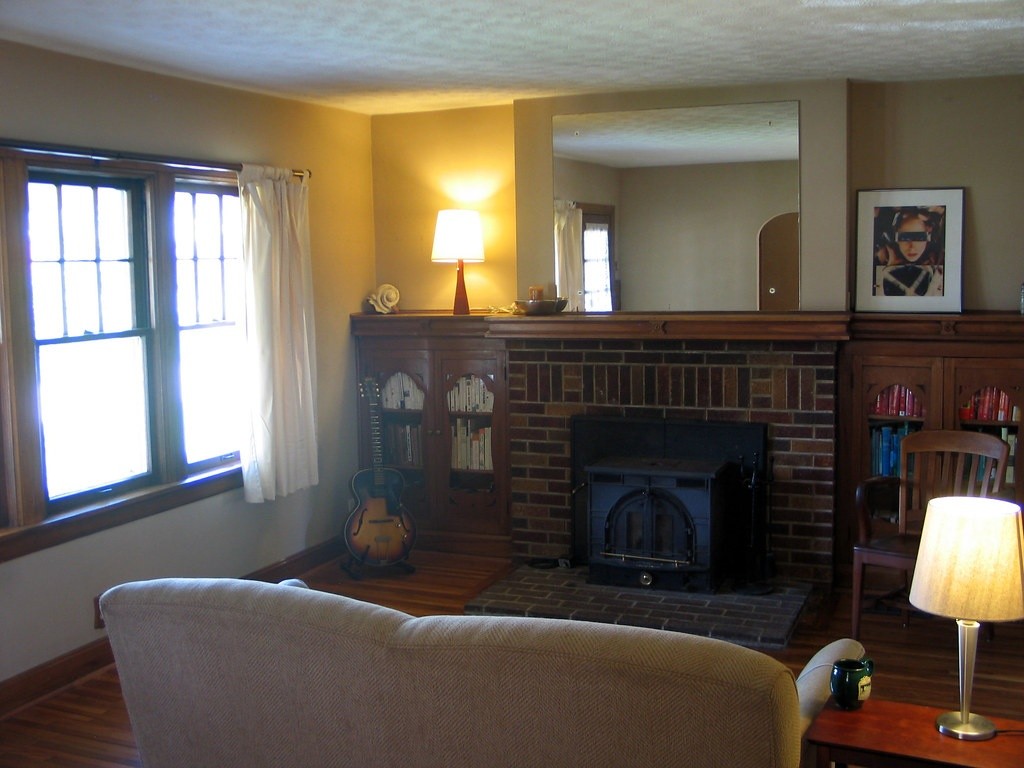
[1020,284,1024,314]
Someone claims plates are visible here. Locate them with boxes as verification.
[516,300,568,315]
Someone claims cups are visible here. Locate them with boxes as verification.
[829,658,874,709]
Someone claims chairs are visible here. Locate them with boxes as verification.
[849,429,1010,642]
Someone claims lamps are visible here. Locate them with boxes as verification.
[909,496,1024,742]
[430,207,487,314]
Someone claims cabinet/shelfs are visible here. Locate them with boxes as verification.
[838,313,1023,592]
[347,308,510,556]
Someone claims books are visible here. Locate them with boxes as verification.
[449,419,493,471]
[869,384,922,417]
[445,375,493,412]
[965,427,1016,483]
[383,423,423,465]
[870,422,915,477]
[959,386,1020,421]
[379,371,424,409]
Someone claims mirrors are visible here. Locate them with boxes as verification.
[551,99,800,312]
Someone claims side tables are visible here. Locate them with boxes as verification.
[803,692,1023,768]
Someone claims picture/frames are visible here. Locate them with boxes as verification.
[856,188,966,312]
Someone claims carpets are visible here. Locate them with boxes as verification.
[464,559,814,650]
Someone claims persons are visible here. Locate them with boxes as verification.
[874,207,945,265]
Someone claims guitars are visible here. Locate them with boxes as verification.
[342,375,418,568]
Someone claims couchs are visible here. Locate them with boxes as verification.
[99,577,867,768]
